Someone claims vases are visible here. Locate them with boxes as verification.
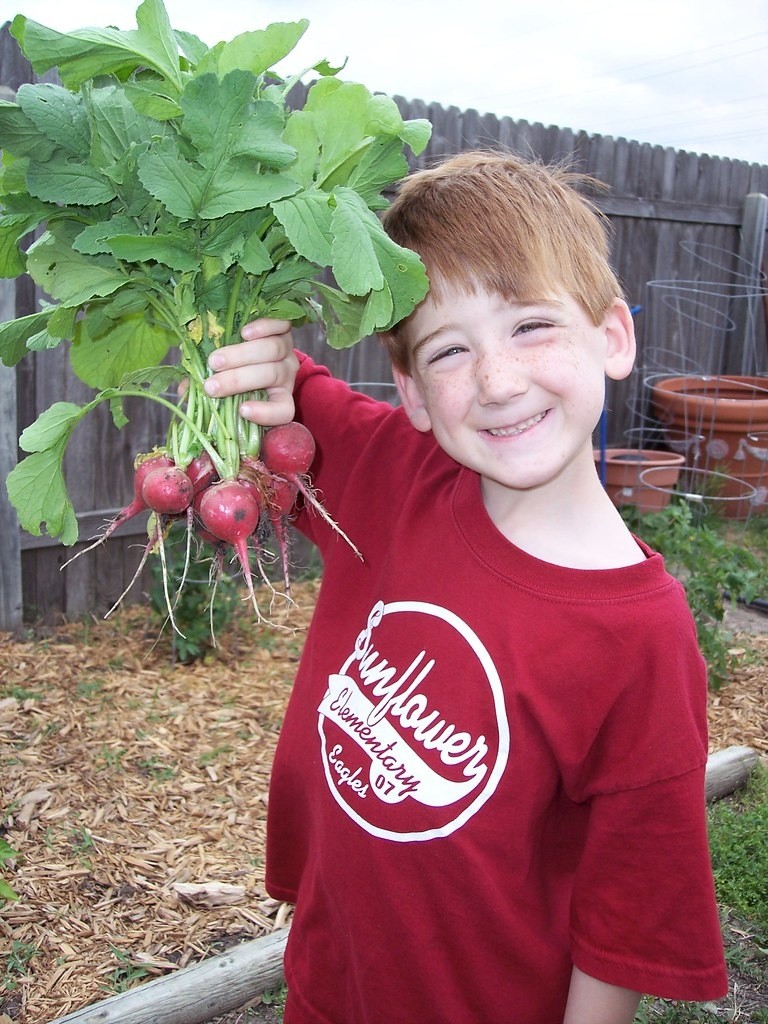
[595,449,685,517]
[650,376,768,523]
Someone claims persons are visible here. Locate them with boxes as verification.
[177,152,728,1024]
